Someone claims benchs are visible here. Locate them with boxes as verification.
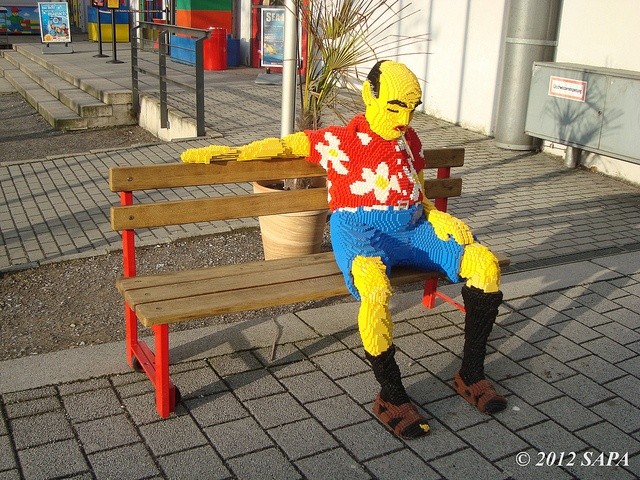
[108,147,466,419]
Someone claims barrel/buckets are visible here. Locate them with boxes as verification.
[226,38,241,66]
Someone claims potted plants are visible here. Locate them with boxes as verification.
[251,0,435,261]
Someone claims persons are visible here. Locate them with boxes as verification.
[180,60,507,439]
